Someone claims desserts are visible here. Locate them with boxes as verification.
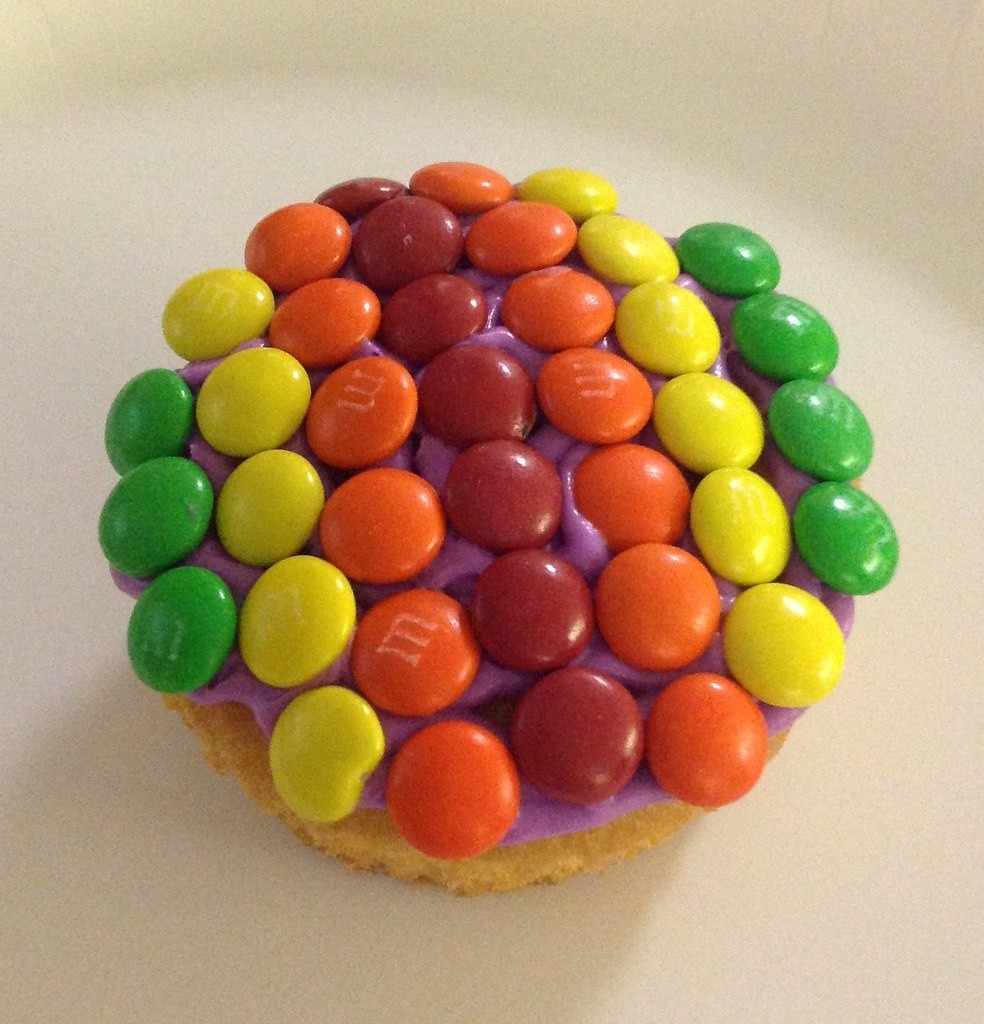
[95,162,898,893]
